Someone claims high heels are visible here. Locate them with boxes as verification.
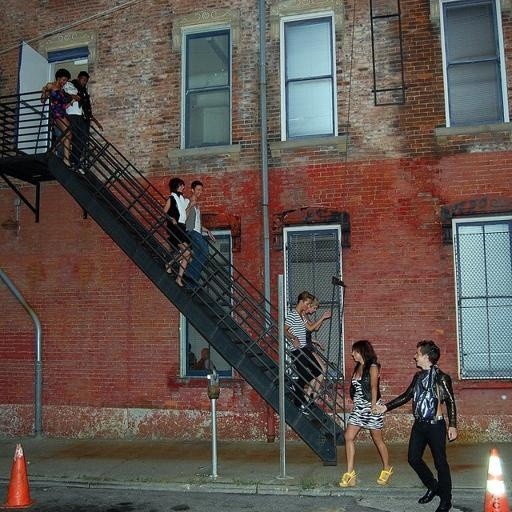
[377,466,394,485]
[339,470,356,488]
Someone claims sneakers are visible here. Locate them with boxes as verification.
[295,404,310,416]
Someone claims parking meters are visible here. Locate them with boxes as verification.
[206,374,221,476]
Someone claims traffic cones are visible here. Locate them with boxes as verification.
[0,444,35,509]
[484,448,510,512]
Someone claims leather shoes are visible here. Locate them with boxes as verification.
[435,503,451,512]
[419,489,440,504]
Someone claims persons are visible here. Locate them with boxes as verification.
[161,178,192,287]
[283,291,330,415]
[185,181,217,289]
[301,298,330,404]
[339,339,394,487]
[41,67,80,166]
[65,71,104,175]
[375,340,459,511]
